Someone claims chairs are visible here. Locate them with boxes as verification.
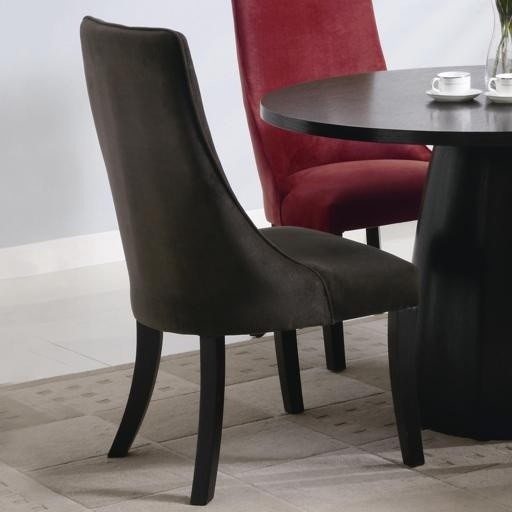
[228,1,428,414]
[78,15,428,502]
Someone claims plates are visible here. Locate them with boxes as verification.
[483,91,512,104]
[425,90,482,103]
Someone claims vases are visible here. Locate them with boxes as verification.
[483,0,512,91]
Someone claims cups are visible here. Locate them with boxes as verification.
[488,74,512,94]
[432,72,472,94]
